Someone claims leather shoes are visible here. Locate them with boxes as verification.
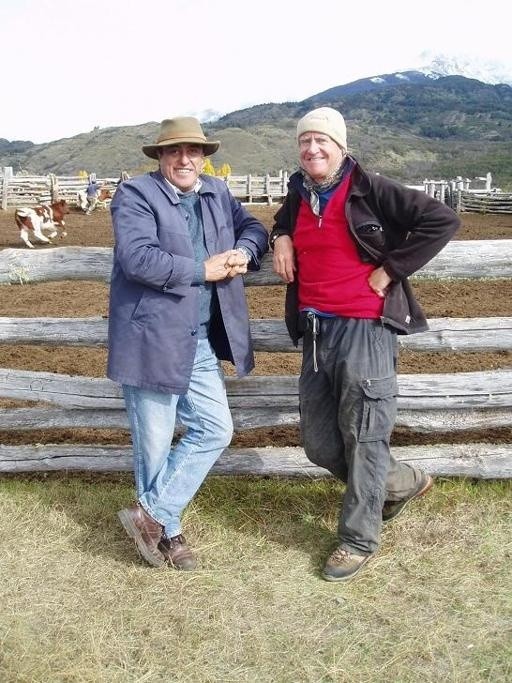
[117,505,196,570]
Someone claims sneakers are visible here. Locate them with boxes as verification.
[321,548,374,581]
[383,470,433,523]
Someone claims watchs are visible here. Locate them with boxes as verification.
[269,233,280,244]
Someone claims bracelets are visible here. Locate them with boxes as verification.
[238,248,252,265]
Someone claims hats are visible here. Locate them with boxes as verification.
[296,107,347,155]
[143,117,220,160]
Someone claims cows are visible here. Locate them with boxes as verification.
[14,198,72,249]
[77,188,114,214]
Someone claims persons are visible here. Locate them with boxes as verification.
[86,181,101,215]
[269,107,463,581]
[106,118,266,570]
